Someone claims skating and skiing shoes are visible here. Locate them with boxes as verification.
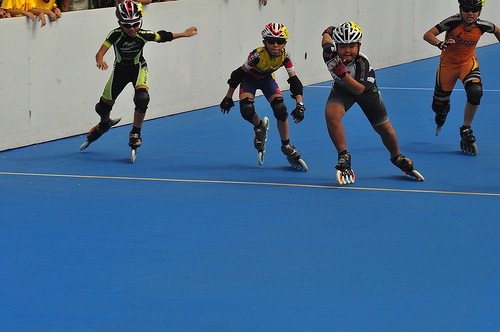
[281,144,308,171]
[80,116,121,151]
[459,126,477,156]
[390,153,424,181]
[435,107,450,136]
[334,151,356,186]
[253,116,269,166]
[129,129,141,162]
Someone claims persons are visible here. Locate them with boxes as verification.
[79,0,197,163]
[261,0,267,6]
[422,0,500,156]
[322,22,424,185]
[219,22,308,171]
[0,0,173,27]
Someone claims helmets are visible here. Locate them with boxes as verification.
[261,22,289,40]
[116,0,143,21]
[458,0,484,7]
[332,22,362,44]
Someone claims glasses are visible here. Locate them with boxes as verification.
[461,7,478,13]
[119,21,141,28]
[266,39,286,45]
